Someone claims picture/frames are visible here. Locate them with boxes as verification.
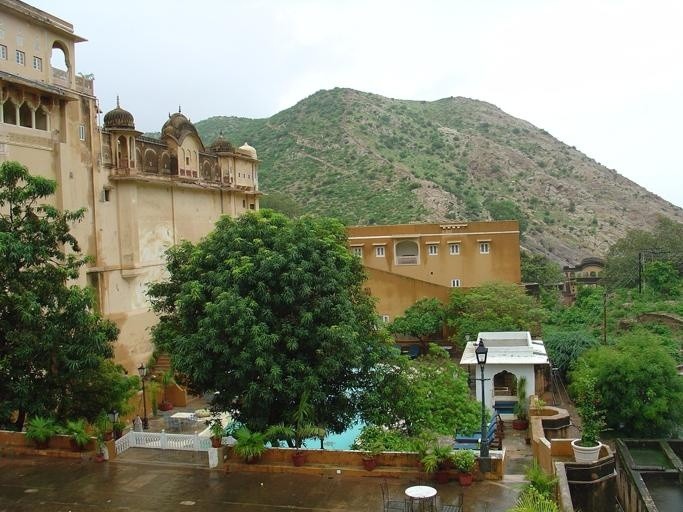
[172,412,195,433]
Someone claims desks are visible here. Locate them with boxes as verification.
[138,363,149,430]
[474,337,494,472]
[109,409,119,443]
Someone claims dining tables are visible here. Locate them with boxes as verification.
[420,441,454,484]
[508,376,529,430]
[267,392,327,467]
[567,362,615,464]
[208,423,226,447]
[452,449,476,486]
[158,369,175,410]
[351,443,388,470]
[25,412,127,462]
[233,426,269,465]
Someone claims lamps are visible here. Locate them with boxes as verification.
[380,480,463,512]
